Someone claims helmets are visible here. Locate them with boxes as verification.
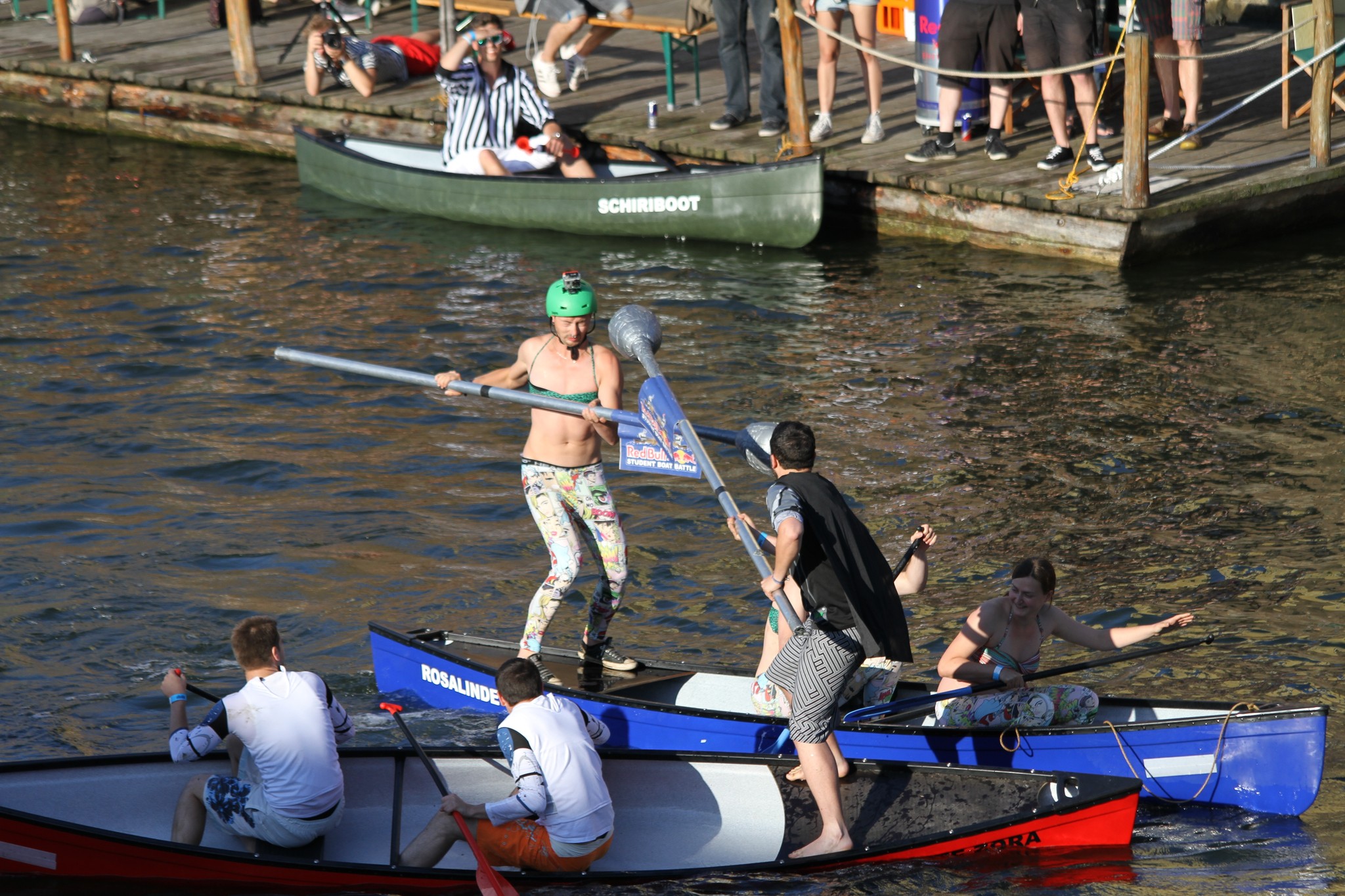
[545,279,599,317]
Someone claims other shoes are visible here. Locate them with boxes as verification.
[809,112,834,142]
[758,120,786,137]
[1178,123,1205,150]
[501,31,518,52]
[326,0,368,22]
[861,115,887,144]
[1147,118,1183,142]
[710,113,748,130]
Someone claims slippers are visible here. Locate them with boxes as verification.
[1052,124,1076,142]
[1094,120,1116,139]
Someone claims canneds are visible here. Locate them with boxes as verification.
[648,102,657,128]
[961,113,972,142]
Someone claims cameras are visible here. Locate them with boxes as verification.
[562,271,582,290]
[321,29,341,49]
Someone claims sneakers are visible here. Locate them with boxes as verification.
[1084,144,1112,171]
[560,47,589,91]
[1037,144,1075,170]
[527,654,563,687]
[578,639,638,671]
[905,138,960,162]
[984,134,1012,160]
[533,50,561,97]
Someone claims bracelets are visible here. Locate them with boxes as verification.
[168,694,187,704]
[339,55,350,65]
[992,665,1004,681]
[757,532,767,547]
[461,30,476,46]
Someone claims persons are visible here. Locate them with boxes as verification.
[514,0,634,99]
[708,0,786,137]
[1134,0,1207,151]
[751,523,938,723]
[435,12,598,179]
[302,18,441,99]
[160,617,352,846]
[396,657,614,872]
[801,0,885,144]
[432,270,638,686]
[935,557,1194,730]
[905,0,1111,173]
[725,420,914,859]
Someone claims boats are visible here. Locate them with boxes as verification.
[363,615,1329,824]
[291,123,832,251]
[0,742,1147,890]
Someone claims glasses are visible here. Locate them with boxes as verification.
[478,34,503,44]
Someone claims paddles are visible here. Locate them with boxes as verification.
[759,526,934,756]
[844,637,1213,725]
[376,698,520,896]
[162,669,222,703]
